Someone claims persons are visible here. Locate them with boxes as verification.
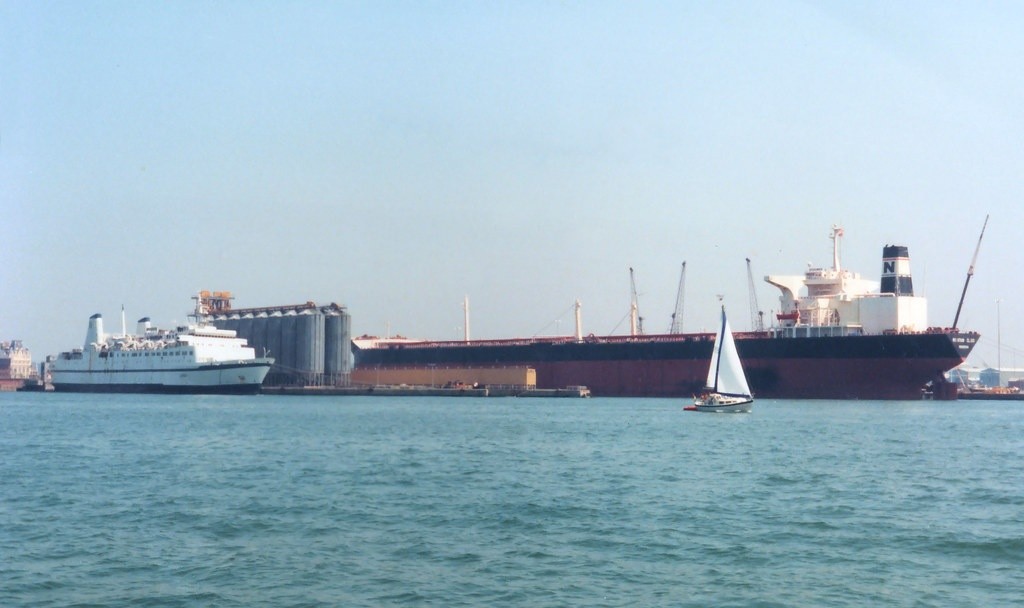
[693,392,708,404]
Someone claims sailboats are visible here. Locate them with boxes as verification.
[694,302,755,415]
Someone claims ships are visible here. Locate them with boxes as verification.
[349,222,980,401]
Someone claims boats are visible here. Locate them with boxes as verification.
[46,292,277,394]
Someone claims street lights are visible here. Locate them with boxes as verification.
[996,298,1001,387]
